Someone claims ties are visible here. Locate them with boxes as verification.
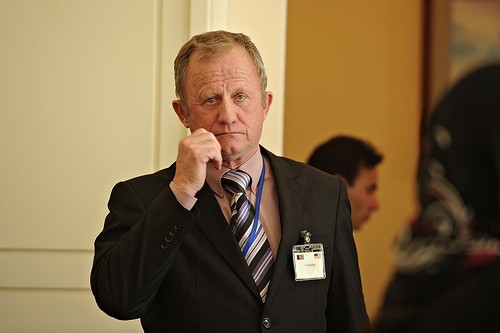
[220,169,274,303]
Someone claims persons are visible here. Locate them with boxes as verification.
[305,133,385,230]
[90,30,371,333]
[373,63,500,333]
